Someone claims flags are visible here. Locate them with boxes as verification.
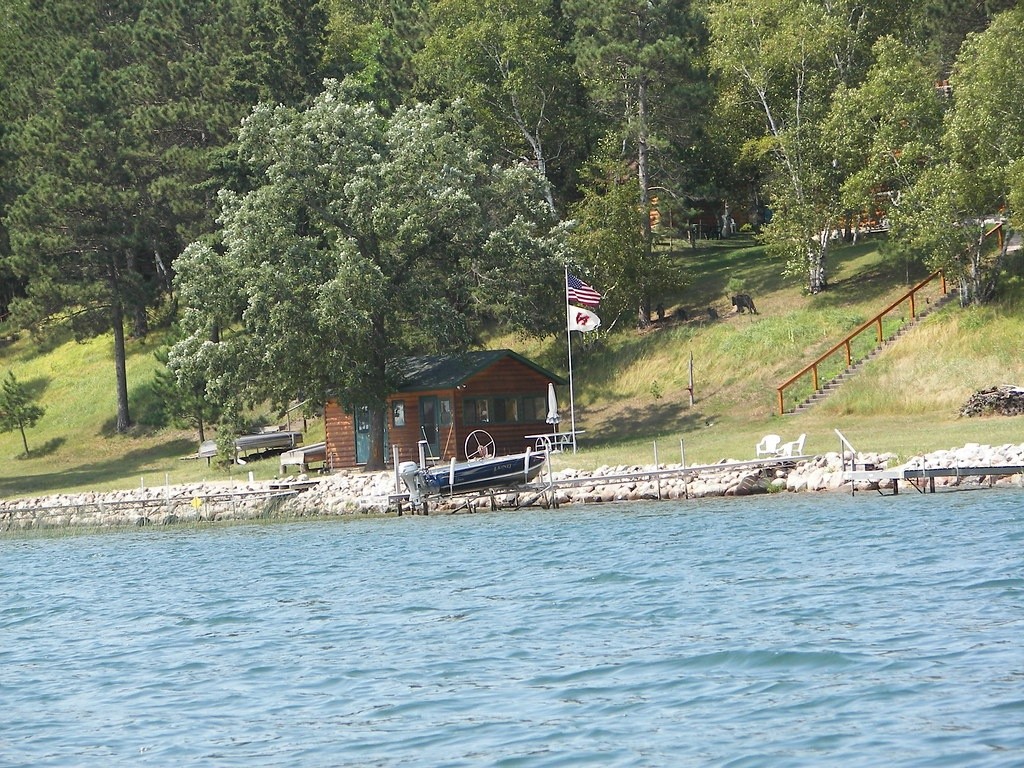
[567,273,601,307]
[569,305,601,332]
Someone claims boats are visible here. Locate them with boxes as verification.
[399,440,547,510]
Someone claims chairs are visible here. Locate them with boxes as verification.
[756,434,780,459]
[775,434,806,458]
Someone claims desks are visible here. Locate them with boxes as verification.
[525,430,586,454]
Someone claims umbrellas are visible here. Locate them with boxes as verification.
[546,383,562,450]
